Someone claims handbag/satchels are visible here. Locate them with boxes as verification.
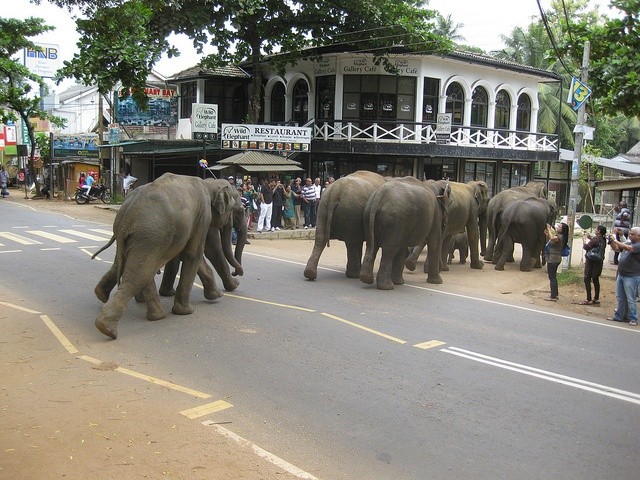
[585,237,604,263]
[562,245,570,256]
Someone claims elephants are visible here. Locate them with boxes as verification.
[448,231,469,265]
[405,179,490,273]
[359,176,452,290]
[90,178,247,303]
[492,197,558,271]
[94,171,244,340]
[304,169,388,280]
[484,181,548,262]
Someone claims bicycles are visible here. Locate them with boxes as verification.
[0,178,11,198]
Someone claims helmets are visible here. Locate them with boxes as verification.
[90,172,95,177]
[80,172,85,177]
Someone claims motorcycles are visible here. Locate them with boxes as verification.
[74,182,111,204]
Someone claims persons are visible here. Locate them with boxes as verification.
[607,226,640,326]
[636,283,640,302]
[122,169,138,200]
[257,178,276,232]
[321,180,330,195]
[309,178,321,227]
[610,201,630,265]
[85,171,100,197]
[288,177,303,228]
[544,222,569,301]
[579,225,606,305]
[0,165,10,198]
[41,173,51,199]
[301,177,317,229]
[271,179,288,232]
[226,175,257,245]
[282,184,304,229]
[612,227,632,312]
[78,172,92,197]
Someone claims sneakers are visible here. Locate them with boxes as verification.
[579,299,592,305]
[305,226,308,229]
[271,227,275,232]
[593,300,600,304]
[629,319,637,326]
[607,317,613,320]
[275,227,281,231]
